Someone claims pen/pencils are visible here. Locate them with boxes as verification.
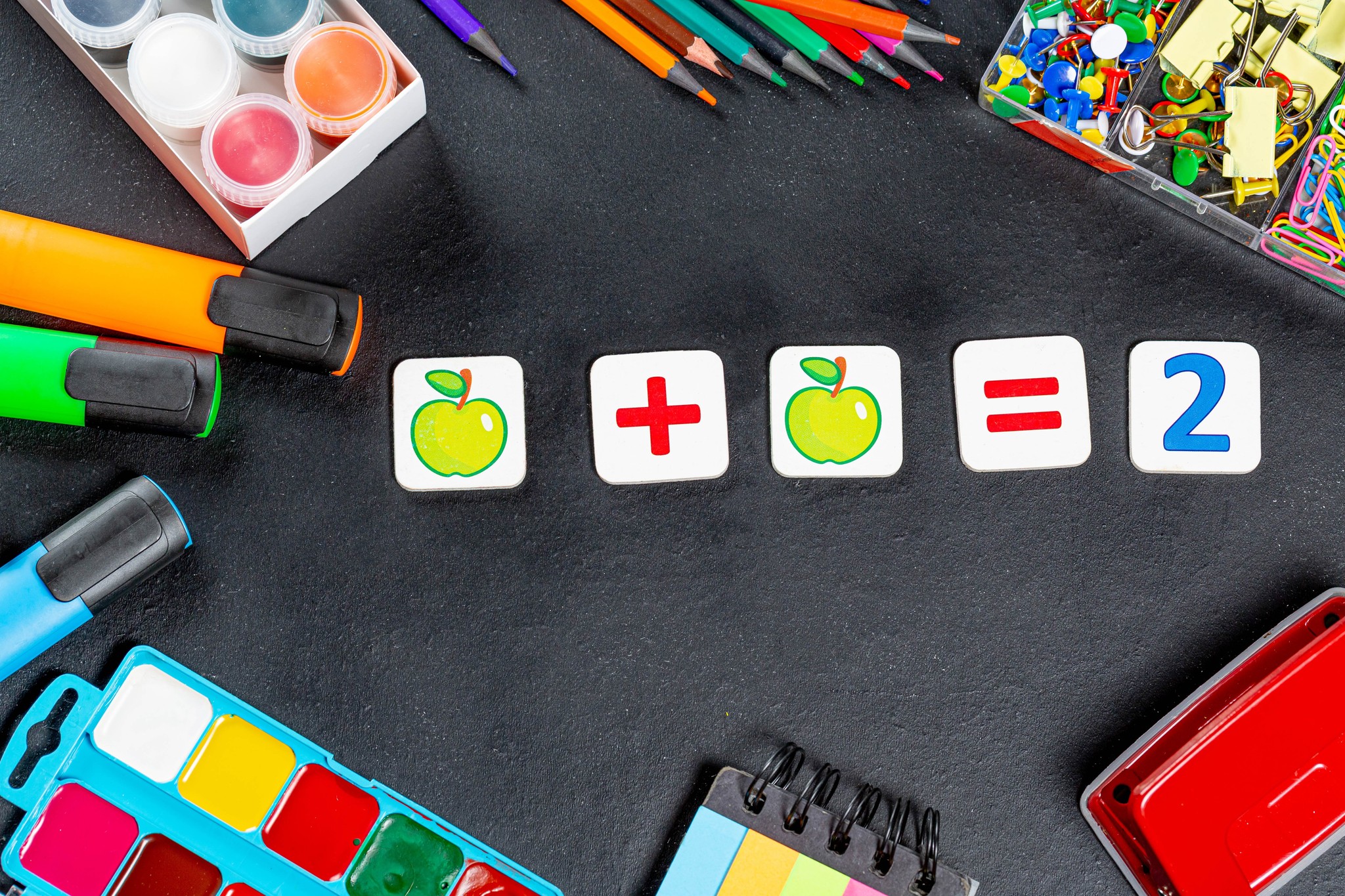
[424,0,517,76]
[563,1,718,106]
[734,0,866,87]
[653,0,789,88]
[694,0,833,92]
[613,0,736,82]
[854,31,945,82]
[745,0,961,45]
[865,0,904,15]
[789,14,911,90]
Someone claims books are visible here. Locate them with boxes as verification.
[656,766,981,896]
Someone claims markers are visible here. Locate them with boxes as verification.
[0,208,364,376]
[0,475,196,686]
[0,322,225,437]
